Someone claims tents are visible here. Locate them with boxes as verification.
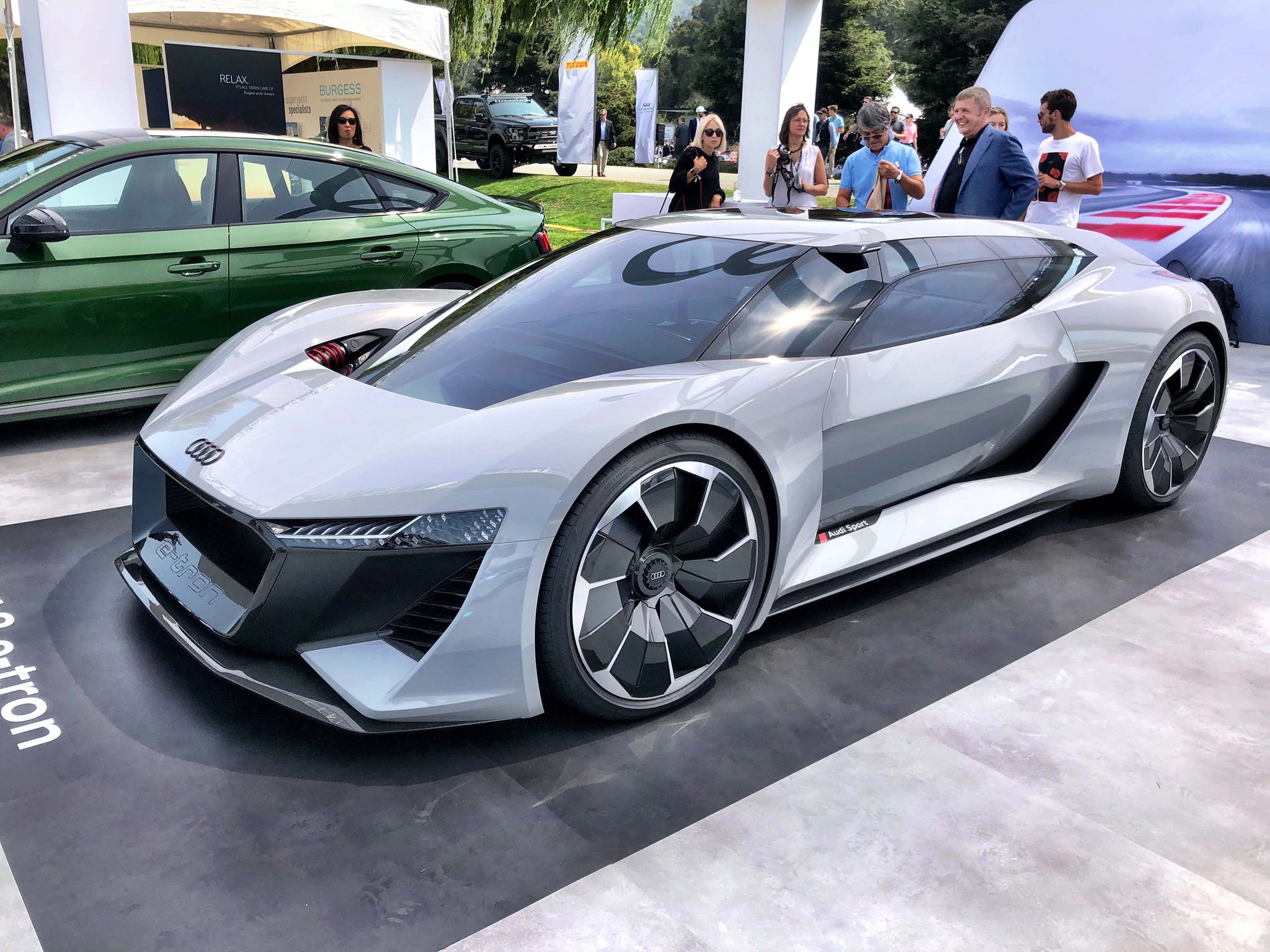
[0,0,458,187]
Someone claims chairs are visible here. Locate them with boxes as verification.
[201,172,214,209]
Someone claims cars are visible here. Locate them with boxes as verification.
[0,126,554,427]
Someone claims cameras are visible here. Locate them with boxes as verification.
[777,147,791,163]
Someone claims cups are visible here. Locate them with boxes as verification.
[1037,171,1045,192]
[733,183,743,203]
[942,128,946,136]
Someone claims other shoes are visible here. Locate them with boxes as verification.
[602,173,606,176]
[598,172,602,176]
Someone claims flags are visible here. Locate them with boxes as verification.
[556,5,594,166]
[634,68,655,164]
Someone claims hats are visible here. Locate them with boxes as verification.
[905,113,913,118]
[816,108,827,114]
[696,106,707,112]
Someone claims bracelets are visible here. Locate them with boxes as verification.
[689,168,702,182]
[765,170,774,177]
[1056,180,1059,189]
[893,170,903,184]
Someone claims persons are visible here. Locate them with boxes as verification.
[763,103,829,206]
[811,96,956,181]
[0,112,35,159]
[673,106,707,160]
[666,112,727,214]
[1020,89,1105,231]
[835,101,925,213]
[313,104,373,152]
[595,109,616,177]
[988,107,1010,132]
[933,86,1039,222]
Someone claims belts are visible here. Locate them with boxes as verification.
[600,139,605,141]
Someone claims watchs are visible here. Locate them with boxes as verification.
[1059,180,1066,190]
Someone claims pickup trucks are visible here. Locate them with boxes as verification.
[434,92,579,179]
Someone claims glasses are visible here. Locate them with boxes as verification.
[905,117,911,119]
[1038,110,1055,120]
[947,107,953,111]
[862,101,868,103]
[860,126,885,140]
[338,117,357,125]
[599,114,605,116]
[818,113,823,115]
[890,111,898,114]
[704,130,723,137]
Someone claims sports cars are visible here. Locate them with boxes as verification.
[113,197,1232,733]
